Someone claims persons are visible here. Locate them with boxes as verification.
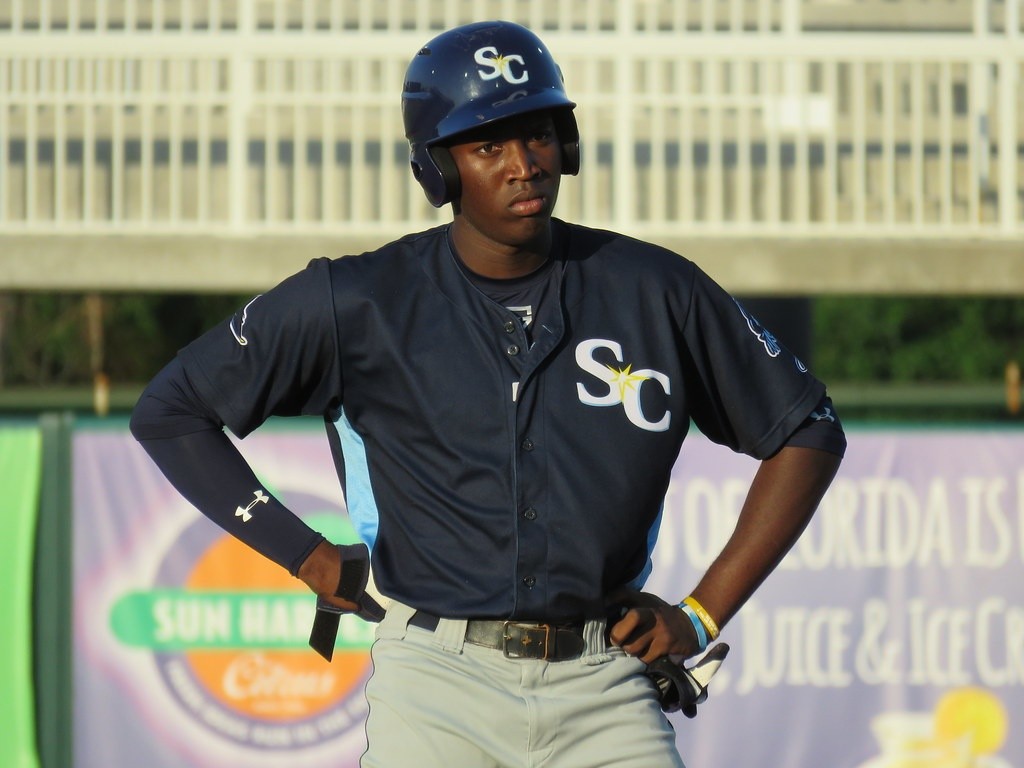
[129,21,847,768]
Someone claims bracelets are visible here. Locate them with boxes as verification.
[674,596,720,654]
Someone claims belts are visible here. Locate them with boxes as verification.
[408,611,613,662]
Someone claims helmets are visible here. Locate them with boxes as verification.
[401,20,581,208]
